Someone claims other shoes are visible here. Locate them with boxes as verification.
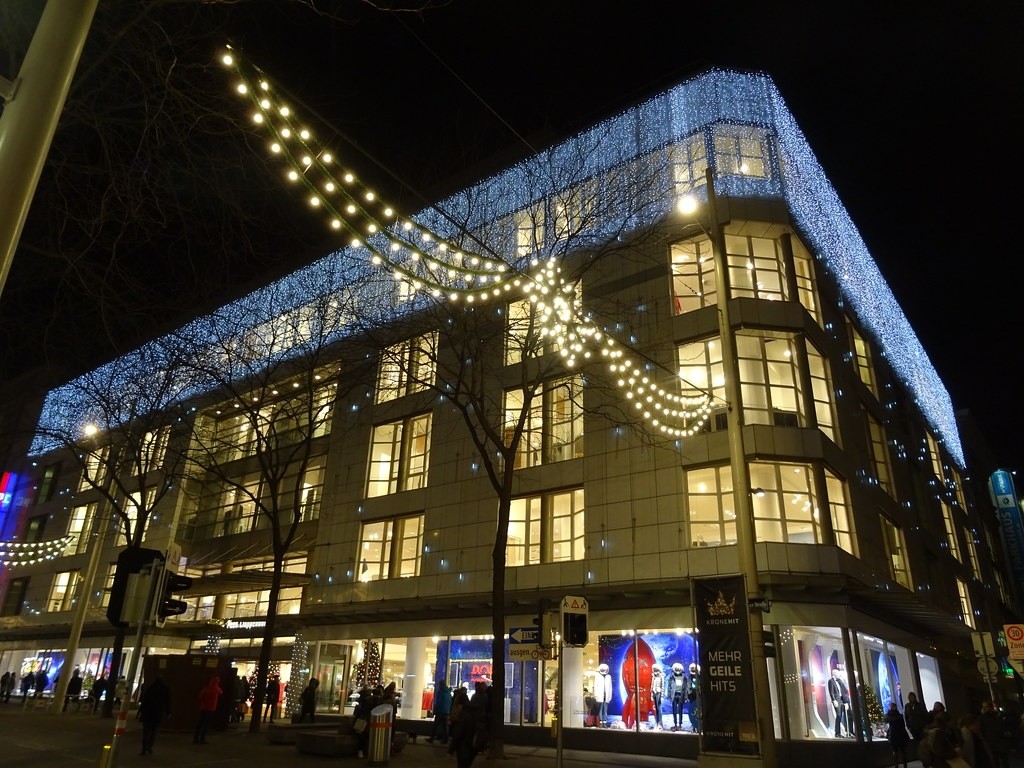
[270,720,275,723]
[138,748,153,756]
[263,720,266,723]
[358,750,364,758]
[426,737,434,742]
[194,740,210,745]
[441,740,448,744]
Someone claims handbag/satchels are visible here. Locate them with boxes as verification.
[352,718,368,735]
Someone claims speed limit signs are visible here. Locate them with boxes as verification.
[1004,623,1024,660]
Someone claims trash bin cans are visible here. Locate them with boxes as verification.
[369,703,393,768]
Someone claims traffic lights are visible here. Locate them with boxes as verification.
[531,612,556,648]
[155,571,191,627]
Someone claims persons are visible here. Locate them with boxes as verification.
[231,675,278,723]
[0,668,126,712]
[300,678,319,723]
[594,663,700,732]
[193,676,223,745]
[828,669,861,739]
[883,692,1009,768]
[135,675,171,755]
[352,682,401,759]
[425,680,493,768]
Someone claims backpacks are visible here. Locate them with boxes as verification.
[463,714,490,748]
